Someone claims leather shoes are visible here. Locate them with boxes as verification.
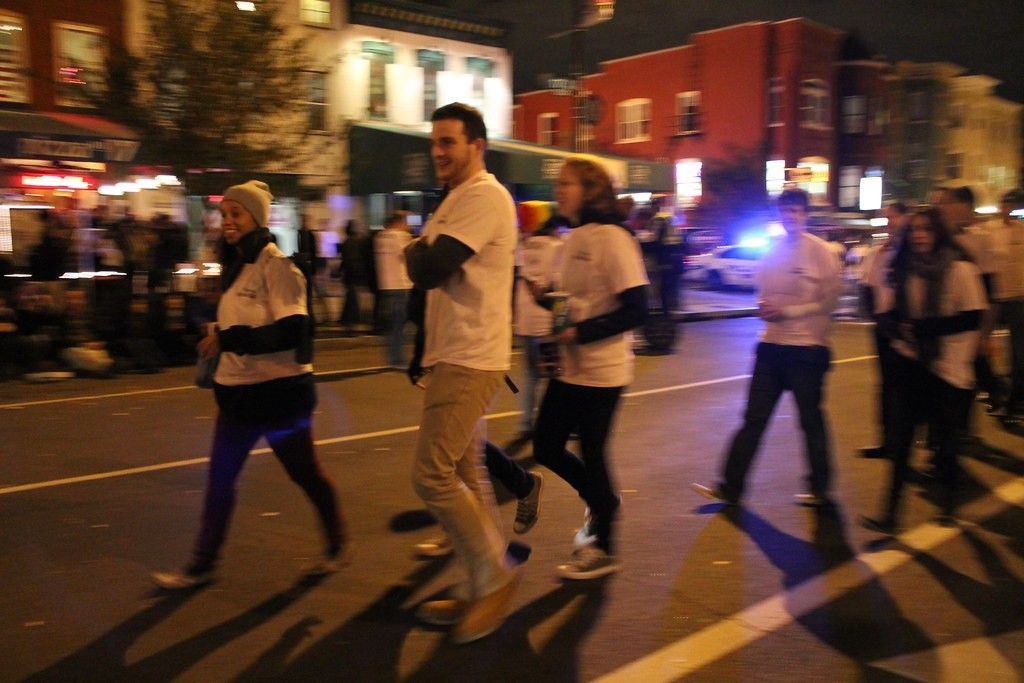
[451,565,527,643]
[420,598,474,623]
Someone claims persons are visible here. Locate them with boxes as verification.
[147,182,357,589]
[523,158,652,577]
[403,101,524,643]
[0,192,685,371]
[849,183,1024,533]
[692,188,844,507]
[413,290,542,556]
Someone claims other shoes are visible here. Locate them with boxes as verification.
[152,572,214,591]
[694,483,739,505]
[861,445,885,456]
[300,537,352,575]
[857,514,895,534]
[792,493,838,503]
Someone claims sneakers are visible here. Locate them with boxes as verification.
[572,493,623,549]
[555,548,623,577]
[512,471,542,533]
[414,532,455,555]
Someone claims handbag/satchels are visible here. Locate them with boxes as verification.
[195,351,218,388]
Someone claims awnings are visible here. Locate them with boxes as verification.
[346,117,674,195]
[1,105,209,166]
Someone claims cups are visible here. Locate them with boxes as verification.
[547,291,570,336]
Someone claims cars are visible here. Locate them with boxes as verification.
[681,244,763,291]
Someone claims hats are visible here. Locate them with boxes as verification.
[224,180,273,227]
